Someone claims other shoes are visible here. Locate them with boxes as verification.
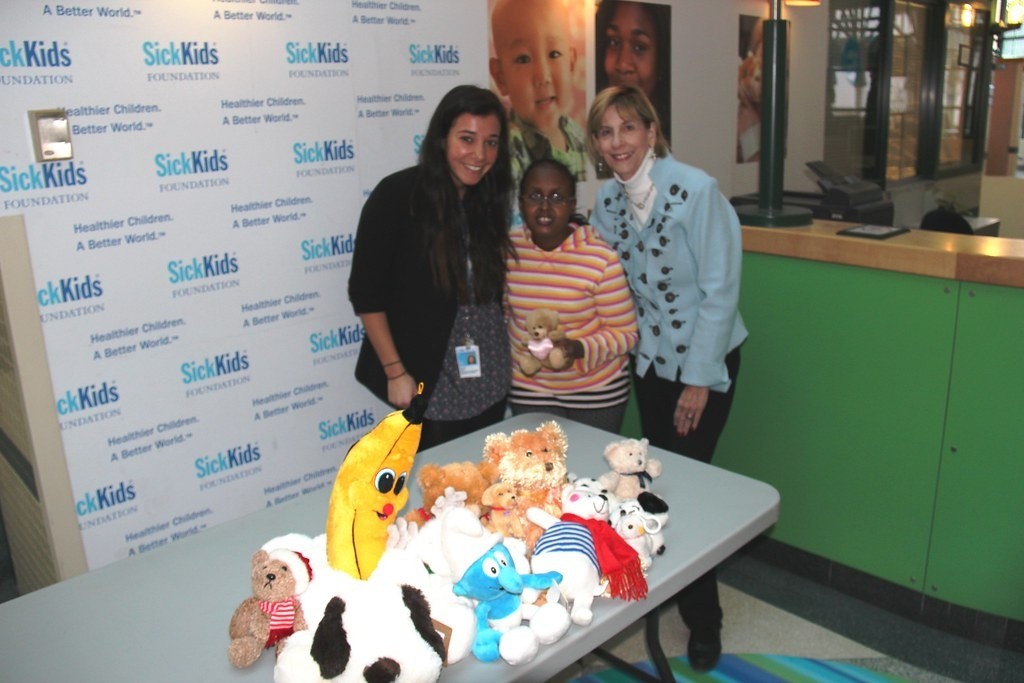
[689,623,720,670]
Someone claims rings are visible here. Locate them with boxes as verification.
[686,414,693,421]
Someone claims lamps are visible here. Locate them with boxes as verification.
[1004,0,1024,29]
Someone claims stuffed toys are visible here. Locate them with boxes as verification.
[225,381,672,683]
[518,306,572,376]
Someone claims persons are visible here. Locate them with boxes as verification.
[343,82,515,452]
[467,356,476,364]
[595,2,671,148]
[737,17,764,164]
[497,157,640,431]
[489,0,588,227]
[589,82,752,672]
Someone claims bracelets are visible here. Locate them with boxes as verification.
[382,360,407,381]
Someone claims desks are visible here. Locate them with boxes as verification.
[0,412,780,682]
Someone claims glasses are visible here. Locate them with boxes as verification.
[519,191,574,205]
